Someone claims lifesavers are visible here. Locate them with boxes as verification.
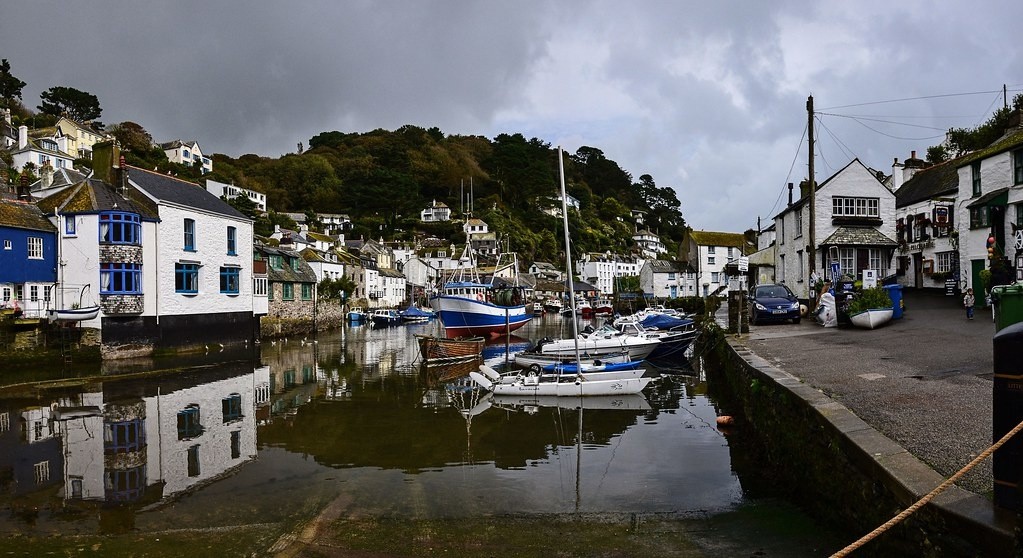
[476,292,484,303]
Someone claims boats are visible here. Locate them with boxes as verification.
[427,174,533,342]
[541,357,645,371]
[536,322,661,363]
[534,297,698,358]
[400,306,436,323]
[513,350,629,370]
[346,306,366,320]
[468,365,651,395]
[45,306,100,320]
[419,335,485,360]
[849,307,893,329]
[373,309,400,324]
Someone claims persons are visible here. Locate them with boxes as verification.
[988,266,1001,322]
[964,288,975,319]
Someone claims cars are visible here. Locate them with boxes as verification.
[746,283,801,324]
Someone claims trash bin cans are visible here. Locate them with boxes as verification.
[881,284,904,320]
[991,283,1023,334]
[993,321,1022,509]
